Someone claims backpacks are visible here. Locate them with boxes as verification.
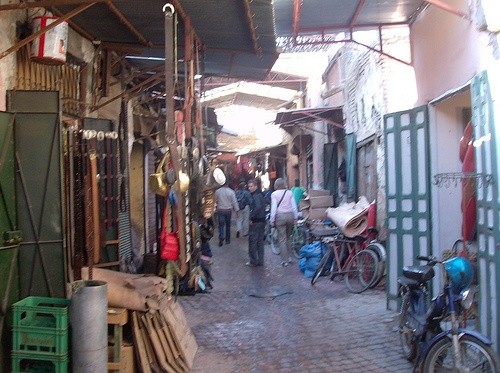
[234,190,249,211]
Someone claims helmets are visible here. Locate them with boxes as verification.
[443,255,474,296]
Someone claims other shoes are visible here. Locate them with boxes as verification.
[218,237,232,247]
[244,260,264,268]
[235,231,240,237]
[279,257,294,268]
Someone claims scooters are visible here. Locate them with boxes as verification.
[391,250,500,373]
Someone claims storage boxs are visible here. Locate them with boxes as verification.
[10,296,72,373]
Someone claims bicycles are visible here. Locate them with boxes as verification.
[311,228,378,293]
[330,232,388,289]
[270,220,310,259]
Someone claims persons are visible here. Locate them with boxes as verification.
[270,178,298,267]
[214,177,239,247]
[244,180,267,266]
[235,181,253,239]
[289,178,306,214]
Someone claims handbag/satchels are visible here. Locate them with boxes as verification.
[213,167,228,187]
[158,197,181,262]
[149,148,173,196]
[176,163,192,197]
